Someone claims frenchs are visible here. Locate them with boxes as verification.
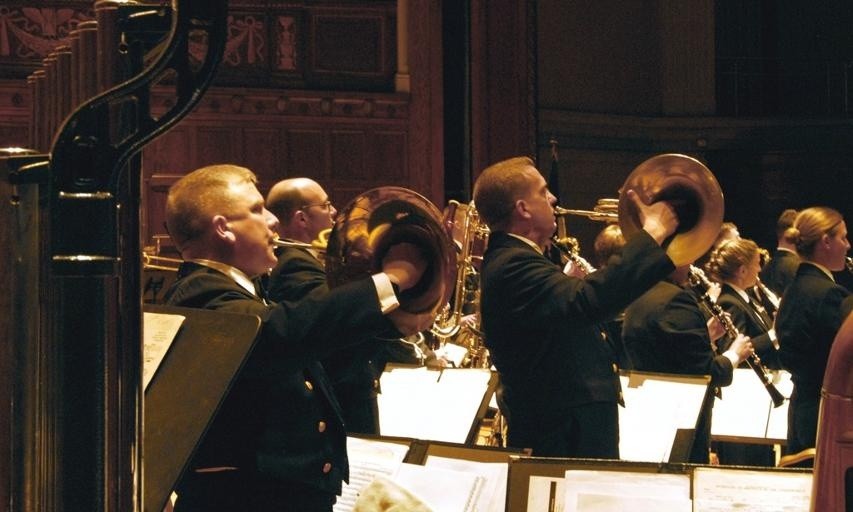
[555,153,724,267]
[270,186,455,339]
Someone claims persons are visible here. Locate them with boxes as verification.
[471,155,694,458]
[772,207,852,466]
[591,206,802,379]
[263,175,388,436]
[163,164,448,512]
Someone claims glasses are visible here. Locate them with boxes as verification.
[288,199,332,213]
